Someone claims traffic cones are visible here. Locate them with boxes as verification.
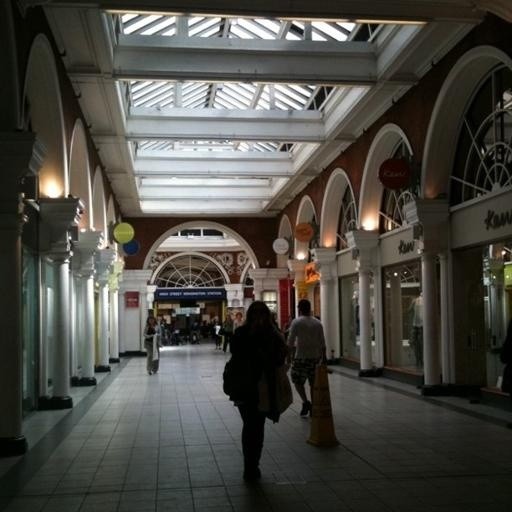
[305,363,341,449]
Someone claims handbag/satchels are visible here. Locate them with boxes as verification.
[223,363,260,406]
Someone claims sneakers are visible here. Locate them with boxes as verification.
[300,400,311,415]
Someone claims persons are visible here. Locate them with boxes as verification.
[285,299,327,418]
[143,316,160,375]
[283,315,297,365]
[407,288,423,367]
[222,300,286,479]
[272,311,280,331]
[155,312,245,352]
